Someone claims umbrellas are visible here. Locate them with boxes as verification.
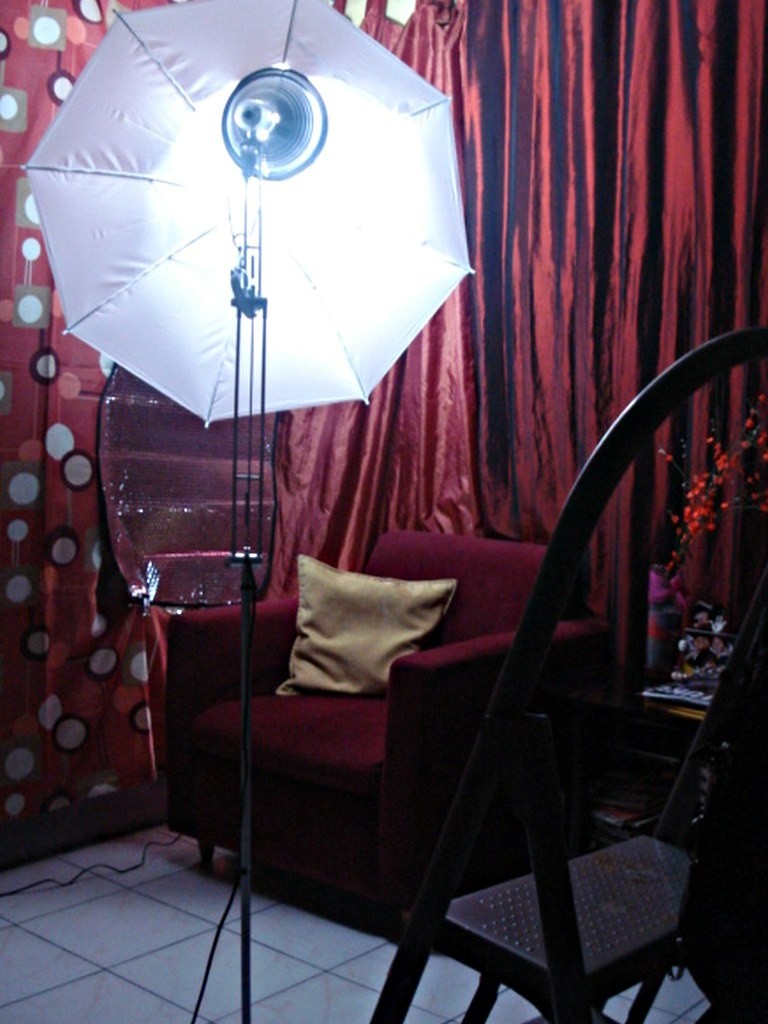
[19,0,477,430]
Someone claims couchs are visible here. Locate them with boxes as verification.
[158,527,614,937]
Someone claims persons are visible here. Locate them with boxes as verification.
[684,612,734,670]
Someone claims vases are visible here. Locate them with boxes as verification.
[646,561,685,686]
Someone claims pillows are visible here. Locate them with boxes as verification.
[276,553,459,700]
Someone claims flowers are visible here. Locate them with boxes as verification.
[657,392,768,578]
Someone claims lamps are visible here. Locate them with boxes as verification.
[17,0,476,1024]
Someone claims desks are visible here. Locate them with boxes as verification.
[567,678,709,849]
